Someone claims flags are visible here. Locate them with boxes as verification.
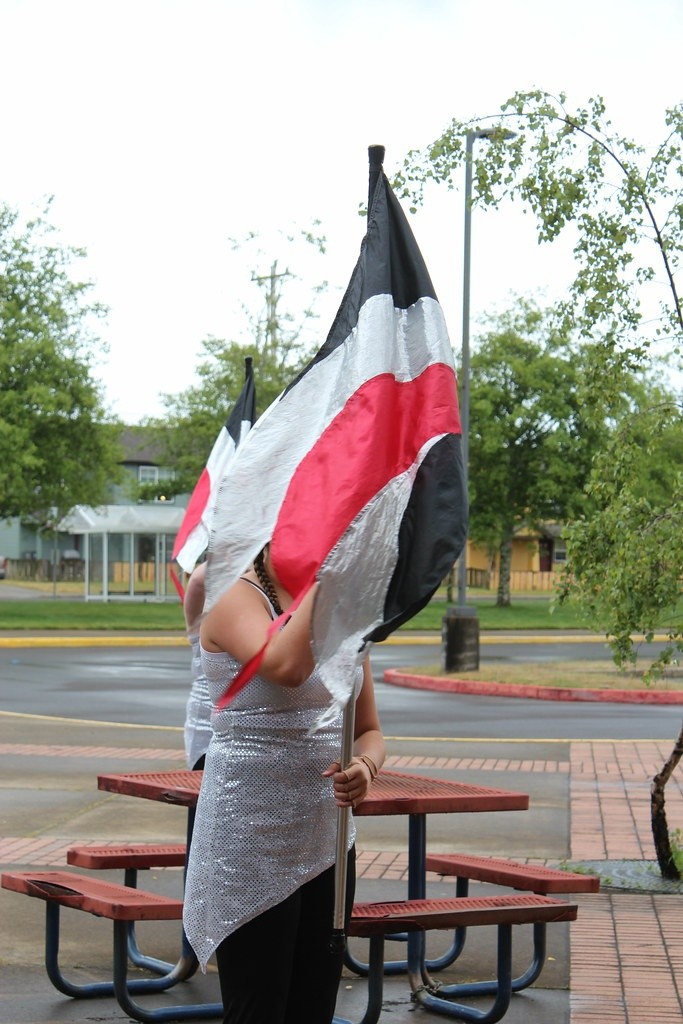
[170,369,258,608]
[199,165,471,715]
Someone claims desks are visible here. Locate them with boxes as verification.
[99,766,531,1023]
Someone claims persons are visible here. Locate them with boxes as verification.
[183,559,214,775]
[182,541,387,1024]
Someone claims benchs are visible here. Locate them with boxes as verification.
[1,870,223,1022]
[67,844,200,981]
[426,851,601,998]
[328,893,578,1024]
